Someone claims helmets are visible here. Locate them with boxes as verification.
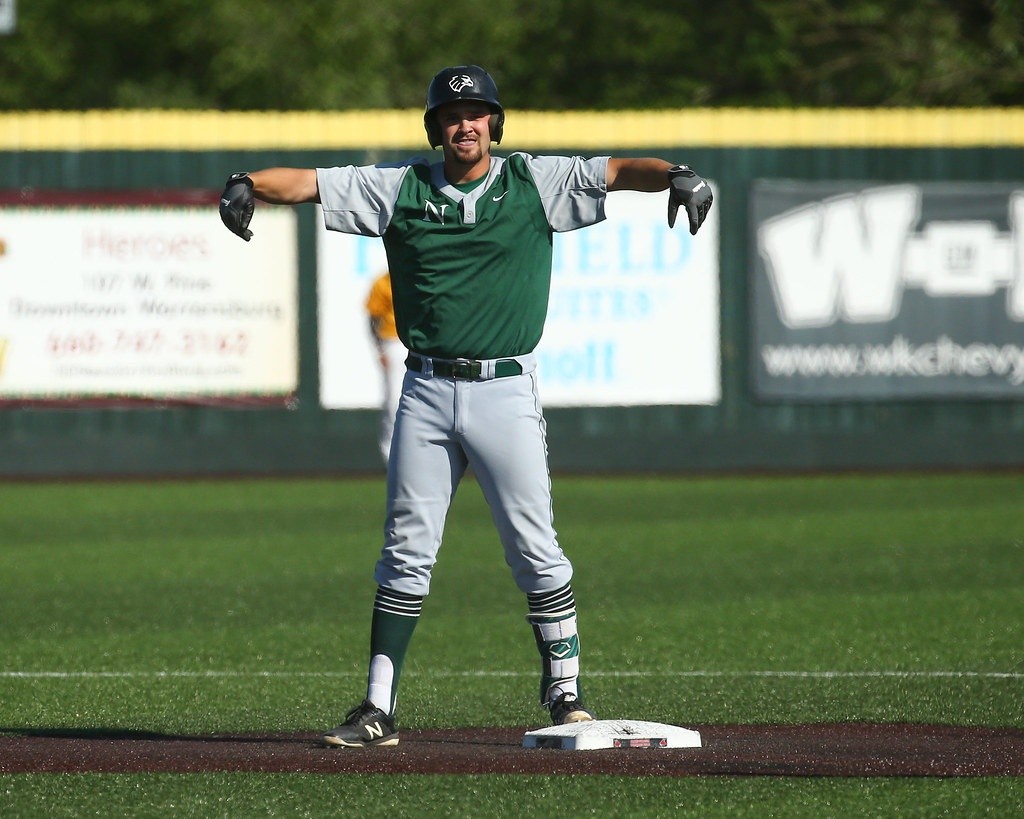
[423,65,505,149]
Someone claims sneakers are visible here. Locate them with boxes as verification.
[541,686,596,726]
[324,699,399,746]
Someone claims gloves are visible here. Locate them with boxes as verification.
[666,163,712,235]
[218,172,254,241]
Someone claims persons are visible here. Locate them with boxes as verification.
[365,272,409,465]
[219,64,714,747]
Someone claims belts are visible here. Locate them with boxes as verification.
[405,353,522,382]
